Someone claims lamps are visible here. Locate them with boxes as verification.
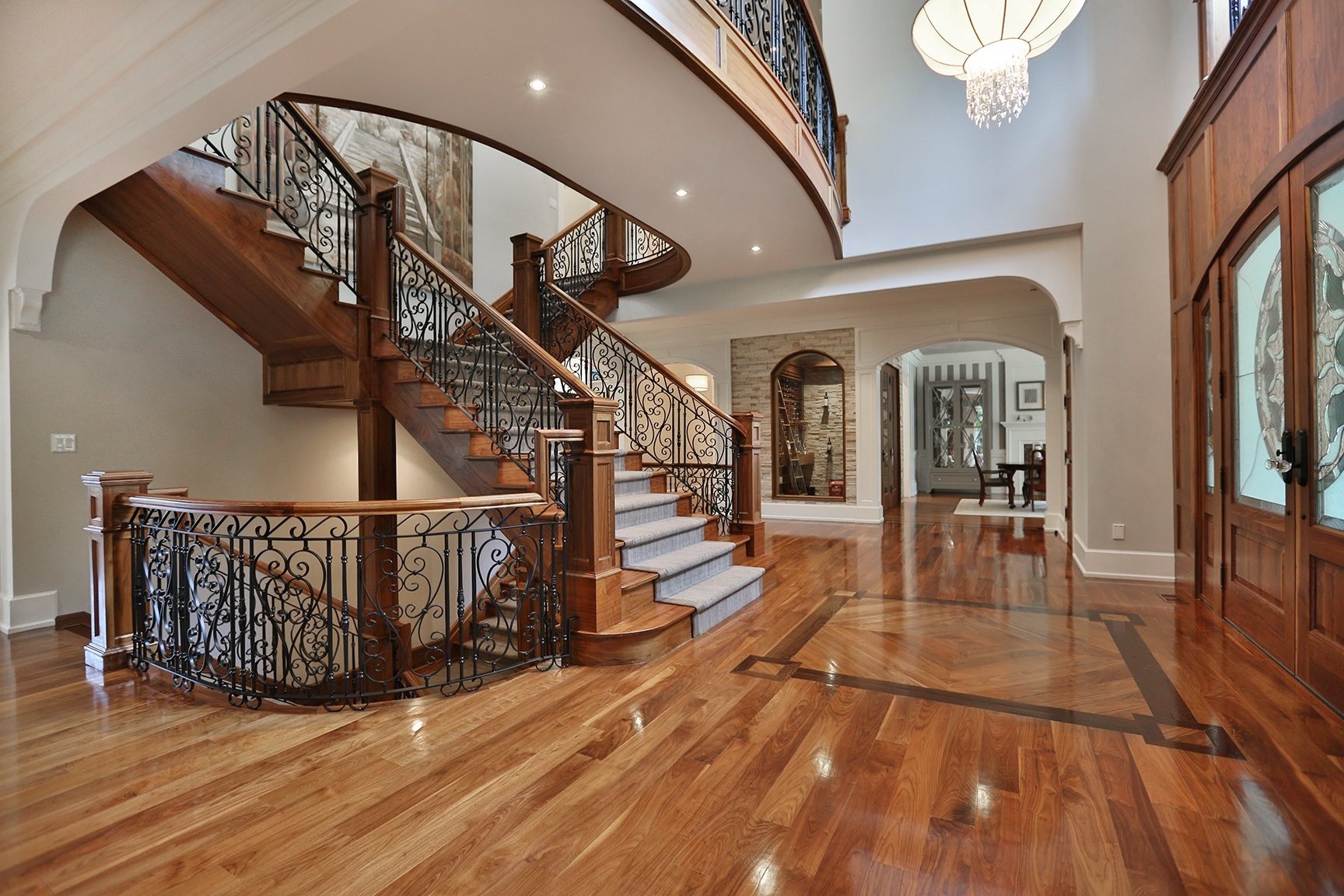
[911,0,1086,130]
[685,374,710,393]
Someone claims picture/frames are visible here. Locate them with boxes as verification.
[1017,381,1045,411]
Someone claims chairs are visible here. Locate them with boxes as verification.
[1019,448,1046,511]
[972,449,1016,509]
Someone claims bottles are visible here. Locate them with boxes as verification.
[824,392,828,406]
[827,437,831,450]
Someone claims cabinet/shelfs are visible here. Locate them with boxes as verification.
[777,376,816,468]
[916,378,990,494]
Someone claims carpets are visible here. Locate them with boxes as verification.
[954,498,1047,518]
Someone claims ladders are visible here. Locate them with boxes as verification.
[778,379,808,496]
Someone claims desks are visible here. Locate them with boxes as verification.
[996,463,1041,509]
